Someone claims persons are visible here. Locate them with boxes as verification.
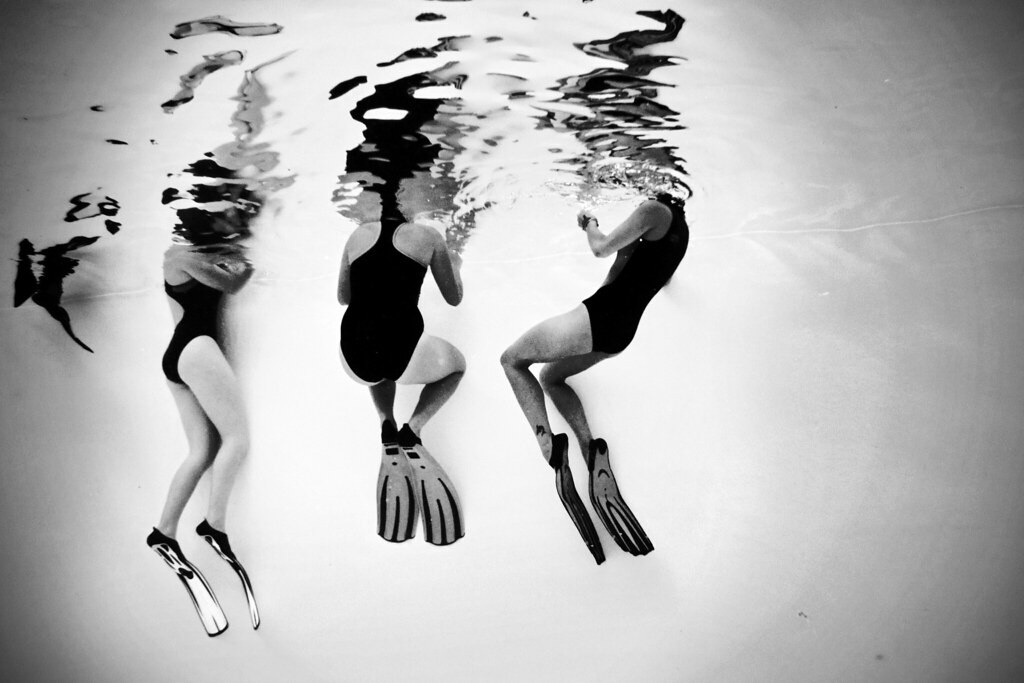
[146,216,265,636]
[338,211,468,544]
[500,196,690,563]
